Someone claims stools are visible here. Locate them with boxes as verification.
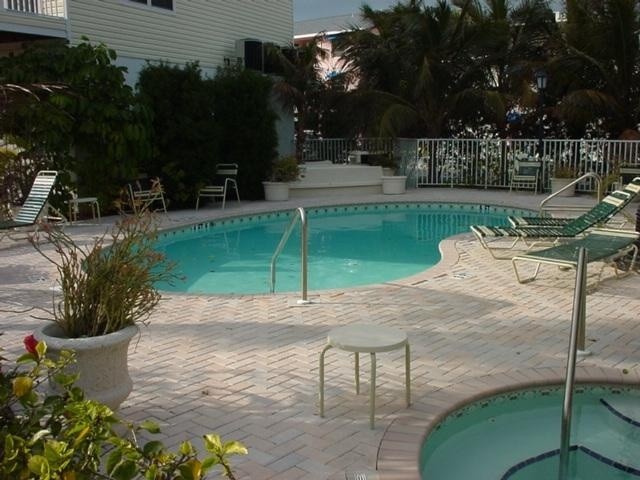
[316,321,414,431]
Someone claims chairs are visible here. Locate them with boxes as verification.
[11,161,243,243]
[466,171,640,295]
[506,157,542,197]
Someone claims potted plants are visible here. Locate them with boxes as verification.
[0,173,189,413]
[260,152,307,202]
[550,163,581,197]
[380,152,407,194]
